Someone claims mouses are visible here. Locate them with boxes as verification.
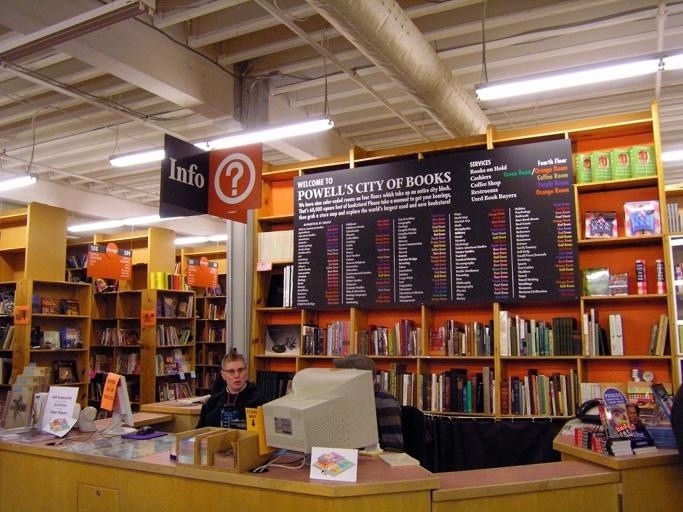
[136,426,153,435]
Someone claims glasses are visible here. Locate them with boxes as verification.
[223,368,247,374]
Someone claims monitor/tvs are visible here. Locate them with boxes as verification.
[101,372,134,434]
[263,367,379,456]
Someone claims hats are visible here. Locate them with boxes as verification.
[333,353,376,375]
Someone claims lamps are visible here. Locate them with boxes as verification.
[108,30,335,168]
[474,0,683,102]
[0,173,40,192]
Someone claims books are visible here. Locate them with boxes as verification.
[571,145,683,458]
[377,452,420,468]
[0,250,226,432]
[264,266,293,307]
[298,304,582,418]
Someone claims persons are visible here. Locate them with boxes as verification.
[194,348,264,430]
[332,353,404,453]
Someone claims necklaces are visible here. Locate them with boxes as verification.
[226,387,239,406]
